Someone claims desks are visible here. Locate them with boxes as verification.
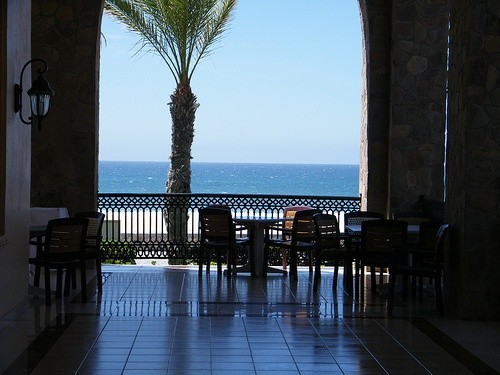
[346,224,420,297]
[28,207,69,292]
[232,218,287,278]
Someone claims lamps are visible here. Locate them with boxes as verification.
[13,58,55,132]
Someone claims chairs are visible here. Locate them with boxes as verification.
[198,205,251,277]
[28,211,105,305]
[264,207,451,317]
